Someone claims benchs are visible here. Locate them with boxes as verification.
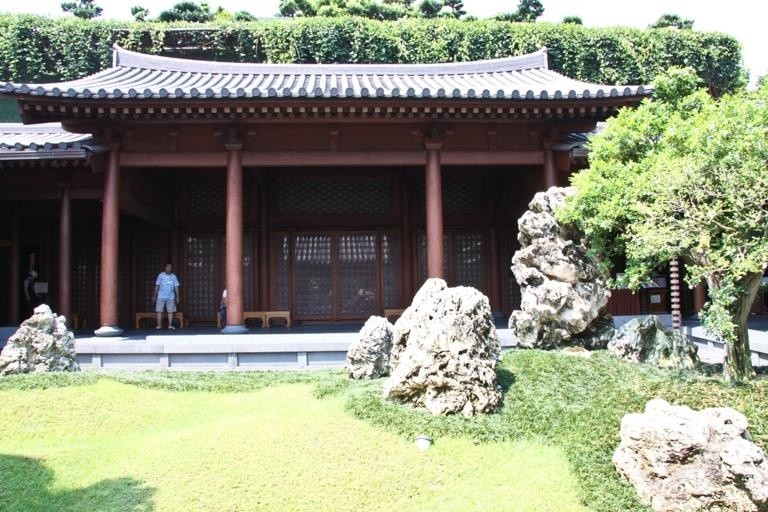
[68,314,79,329]
[223,311,291,328]
[384,309,403,323]
[136,313,188,329]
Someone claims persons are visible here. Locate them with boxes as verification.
[151,263,180,331]
[219,289,227,329]
[22,270,39,319]
